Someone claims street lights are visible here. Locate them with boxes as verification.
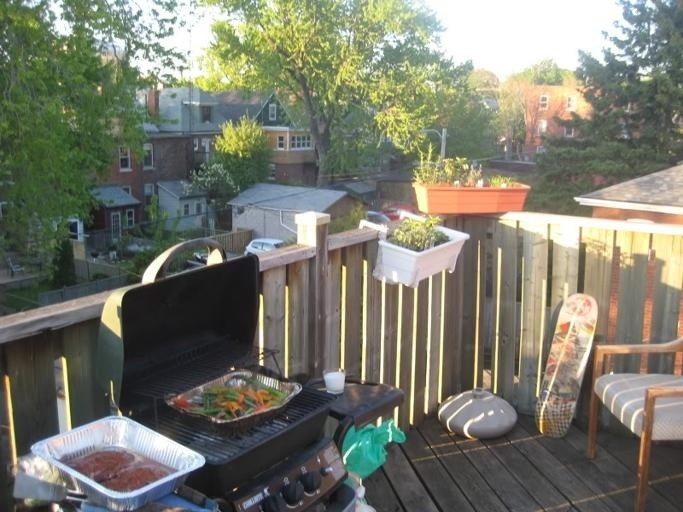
[422,127,448,159]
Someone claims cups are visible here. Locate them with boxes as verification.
[323,367,346,396]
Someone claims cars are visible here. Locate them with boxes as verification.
[366,203,418,224]
[245,238,284,256]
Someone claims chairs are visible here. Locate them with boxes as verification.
[586,335,683,512]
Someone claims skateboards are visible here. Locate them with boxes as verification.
[534,293,598,438]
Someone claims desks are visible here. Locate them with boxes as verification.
[309,377,405,458]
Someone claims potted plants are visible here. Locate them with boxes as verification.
[410,141,531,214]
[371,215,471,288]
